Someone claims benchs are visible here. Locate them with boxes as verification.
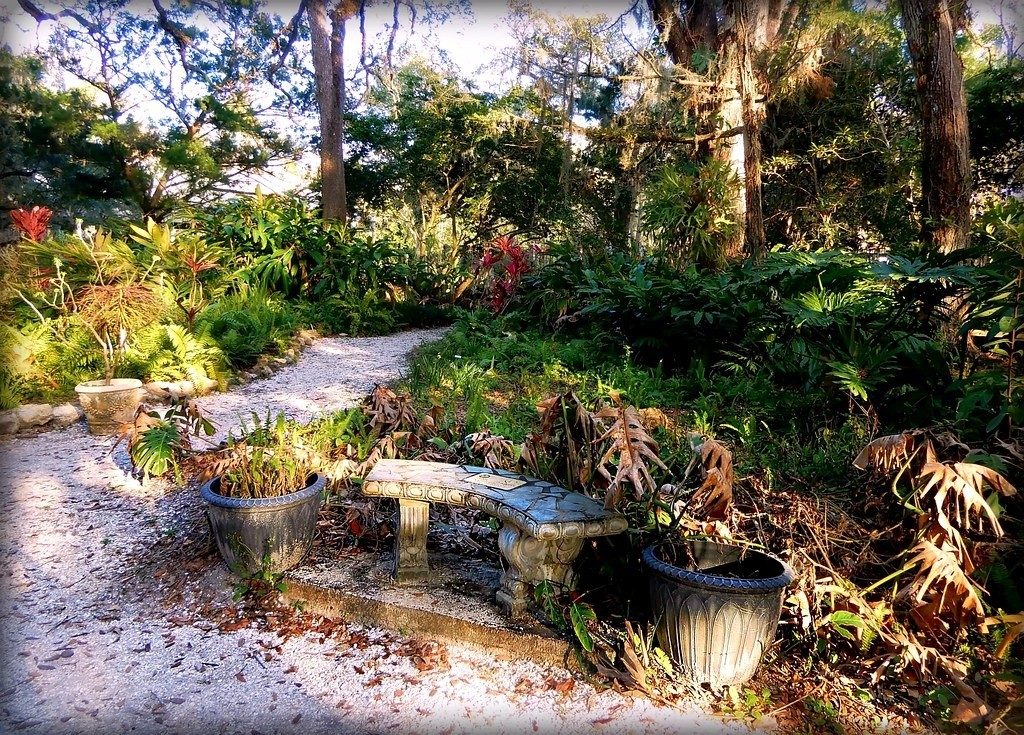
[359,457,630,623]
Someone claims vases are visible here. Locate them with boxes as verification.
[637,538,793,688]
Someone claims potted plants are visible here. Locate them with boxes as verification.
[200,406,327,581]
[37,212,164,439]
